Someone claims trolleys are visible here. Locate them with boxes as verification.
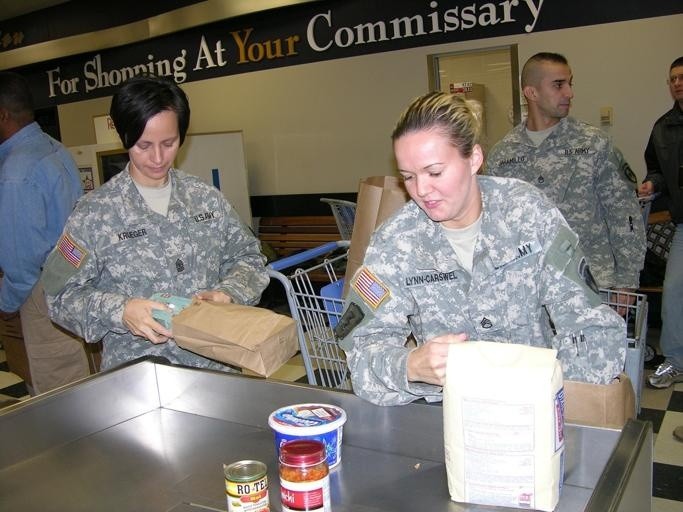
[254,234,649,423]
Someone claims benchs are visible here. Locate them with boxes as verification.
[253,215,347,310]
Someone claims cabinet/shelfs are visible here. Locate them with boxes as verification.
[0,354,655,510]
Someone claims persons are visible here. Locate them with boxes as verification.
[471,47,648,326]
[636,54,683,391]
[0,66,91,400]
[35,69,272,381]
[333,88,628,409]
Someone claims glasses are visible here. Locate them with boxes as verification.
[666,74,683,84]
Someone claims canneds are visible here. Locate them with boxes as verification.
[278,440,332,512]
[223,459,271,512]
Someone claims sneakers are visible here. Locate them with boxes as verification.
[673,425,683,439]
[648,363,683,389]
